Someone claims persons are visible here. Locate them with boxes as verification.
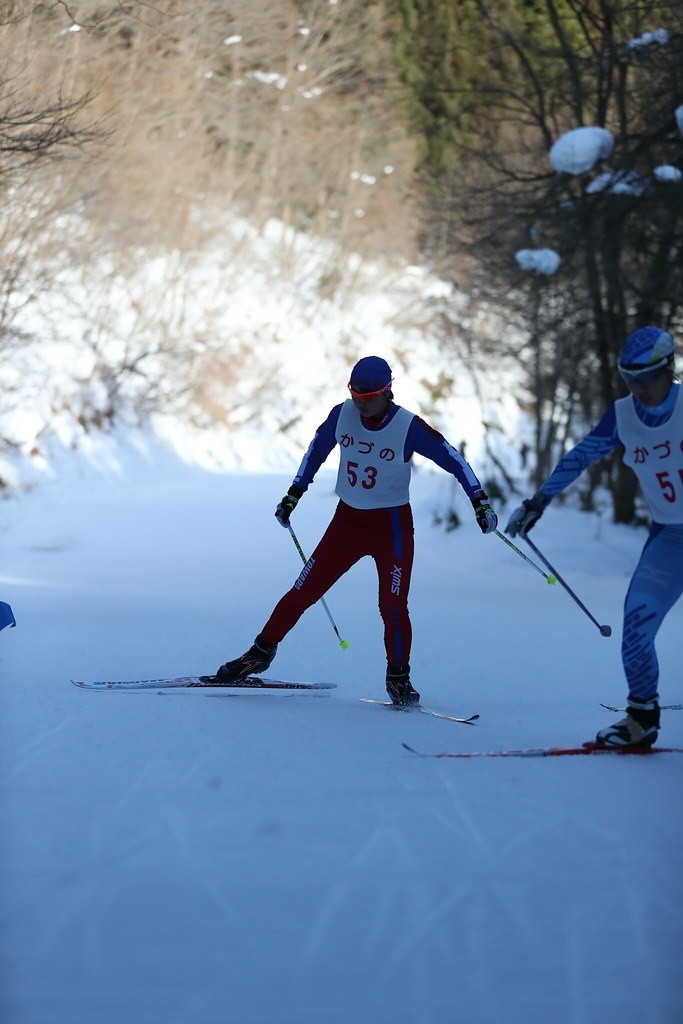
[216,356,499,706]
[504,328,683,754]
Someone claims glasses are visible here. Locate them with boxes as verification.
[347,382,391,401]
[617,355,669,382]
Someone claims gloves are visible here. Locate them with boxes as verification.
[504,498,544,538]
[472,499,498,534]
[276,494,299,529]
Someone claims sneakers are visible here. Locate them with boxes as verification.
[217,634,277,681]
[385,665,420,704]
[596,693,661,747]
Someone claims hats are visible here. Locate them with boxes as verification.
[350,355,392,392]
[620,326,675,368]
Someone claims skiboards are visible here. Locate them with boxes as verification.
[70,677,683,760]
